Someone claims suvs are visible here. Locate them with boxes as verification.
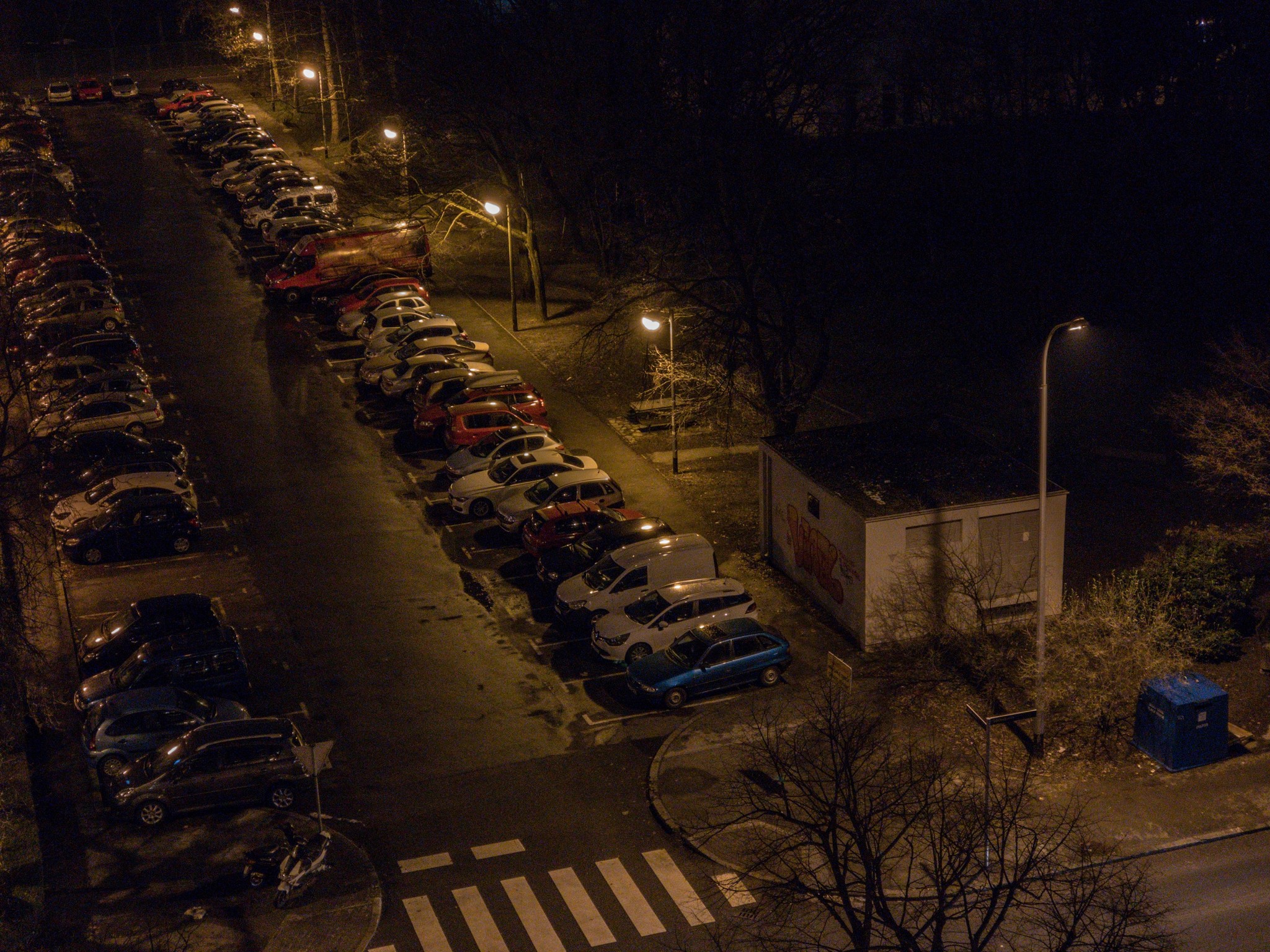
[73,593,310,832]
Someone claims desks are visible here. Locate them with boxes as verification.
[629,396,684,423]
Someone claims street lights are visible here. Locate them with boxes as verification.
[1032,317,1089,759]
[383,125,411,218]
[253,31,275,112]
[484,197,518,332]
[303,66,328,159]
[641,309,678,475]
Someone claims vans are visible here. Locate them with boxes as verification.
[262,218,436,305]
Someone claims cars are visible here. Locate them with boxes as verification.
[73,76,103,102]
[148,77,354,261]
[0,93,203,569]
[309,266,793,711]
[108,73,137,98]
[45,80,73,105]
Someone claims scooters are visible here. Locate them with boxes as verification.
[240,821,333,909]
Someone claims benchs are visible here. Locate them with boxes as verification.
[638,416,682,433]
